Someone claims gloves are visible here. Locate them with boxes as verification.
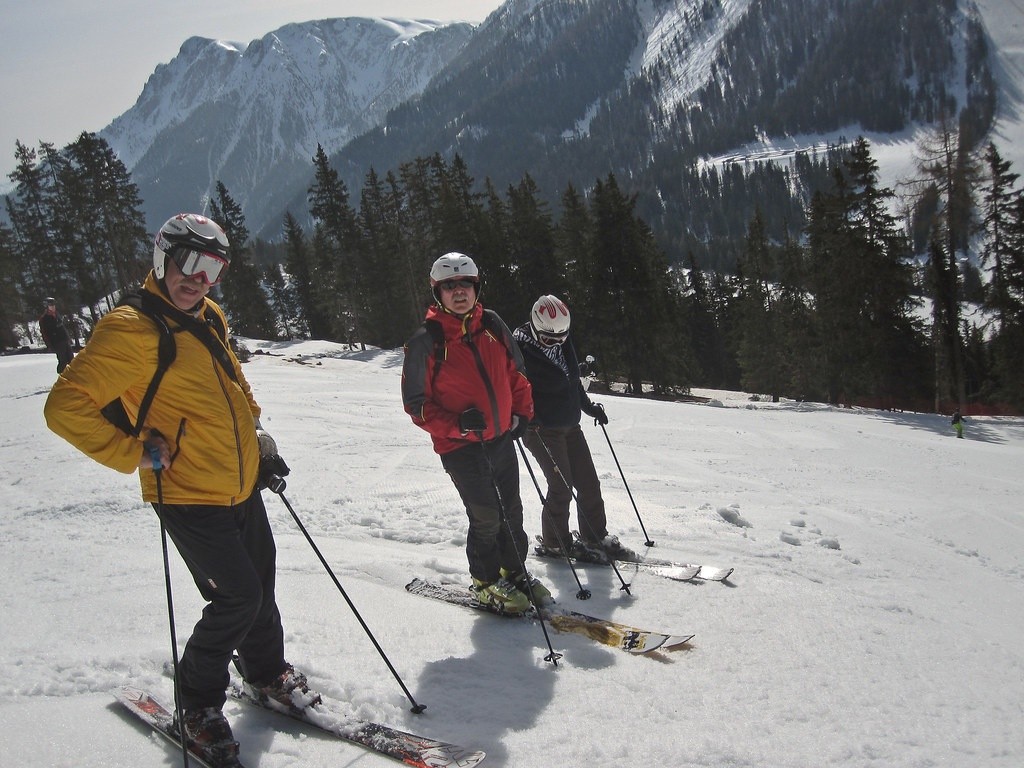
[460,408,488,436]
[527,413,543,432]
[509,414,528,440]
[254,421,278,458]
[593,406,608,425]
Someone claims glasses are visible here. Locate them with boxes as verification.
[171,244,228,286]
[441,278,472,290]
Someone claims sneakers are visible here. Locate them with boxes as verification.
[546,544,602,563]
[241,667,321,711]
[174,706,239,766]
[577,535,636,562]
[471,568,551,615]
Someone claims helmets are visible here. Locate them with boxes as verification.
[43,297,57,308]
[530,295,570,347]
[153,213,232,284]
[429,252,483,305]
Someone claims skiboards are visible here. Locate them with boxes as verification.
[535,530,734,581]
[404,576,694,653]
[110,660,485,768]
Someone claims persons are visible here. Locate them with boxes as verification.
[44,212,308,747]
[512,295,627,566]
[951,407,967,439]
[40,297,74,375]
[401,252,553,614]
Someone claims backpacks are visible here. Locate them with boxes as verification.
[88,291,226,437]
